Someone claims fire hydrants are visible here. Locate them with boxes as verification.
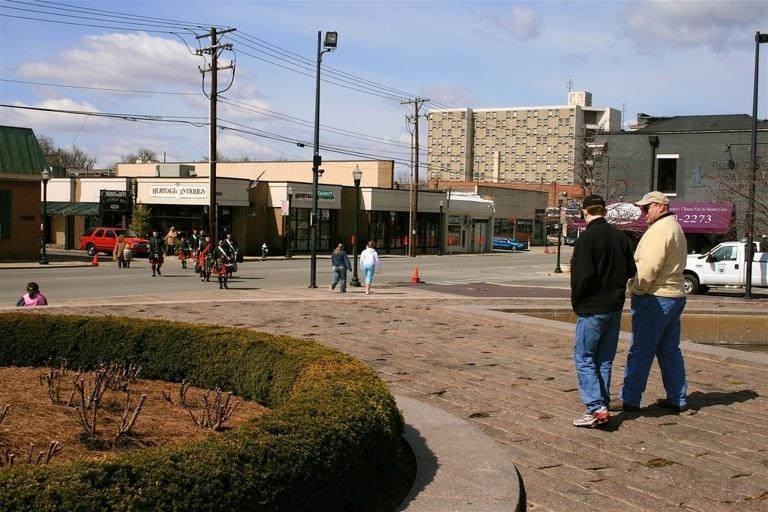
[262,243,269,258]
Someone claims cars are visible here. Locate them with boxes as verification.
[546,234,566,246]
[564,234,582,247]
[495,231,532,242]
[493,236,528,251]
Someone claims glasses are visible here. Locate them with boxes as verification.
[639,205,649,214]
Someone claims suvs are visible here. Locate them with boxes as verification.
[79,227,150,258]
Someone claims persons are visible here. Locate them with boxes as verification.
[123,244,132,268]
[608,191,688,412]
[330,242,352,292]
[147,227,240,289]
[113,235,126,268]
[571,194,637,427]
[360,241,379,295]
[17,282,47,306]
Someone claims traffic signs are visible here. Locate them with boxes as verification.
[318,191,333,197]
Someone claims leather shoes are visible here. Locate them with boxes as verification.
[658,399,687,411]
[608,398,640,410]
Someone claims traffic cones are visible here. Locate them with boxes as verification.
[544,247,549,253]
[91,254,98,266]
[411,266,420,283]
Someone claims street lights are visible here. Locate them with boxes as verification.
[284,186,294,260]
[438,199,444,256]
[743,31,767,300]
[554,192,564,274]
[307,30,338,289]
[39,167,50,264]
[349,164,363,287]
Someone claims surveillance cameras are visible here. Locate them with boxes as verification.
[319,169,324,173]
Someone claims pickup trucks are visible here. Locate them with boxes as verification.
[684,240,768,295]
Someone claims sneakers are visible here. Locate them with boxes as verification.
[573,404,610,427]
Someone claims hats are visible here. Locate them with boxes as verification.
[580,195,605,220]
[634,191,670,207]
